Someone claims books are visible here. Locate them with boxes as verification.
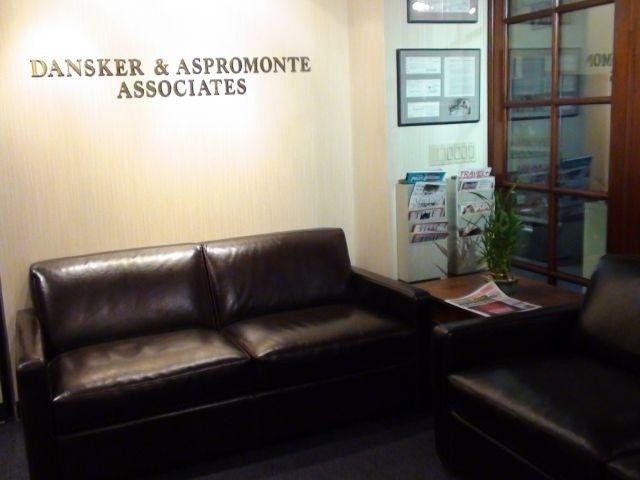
[405,167,495,243]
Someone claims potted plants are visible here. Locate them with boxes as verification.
[461,180,531,294]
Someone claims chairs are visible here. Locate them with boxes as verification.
[433,252,639,480]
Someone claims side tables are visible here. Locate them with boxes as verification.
[409,269,584,423]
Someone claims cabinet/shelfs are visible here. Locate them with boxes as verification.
[445,175,496,275]
[395,181,448,284]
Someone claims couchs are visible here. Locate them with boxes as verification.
[13,226,431,480]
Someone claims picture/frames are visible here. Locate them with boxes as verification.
[407,0,479,23]
[398,48,481,125]
